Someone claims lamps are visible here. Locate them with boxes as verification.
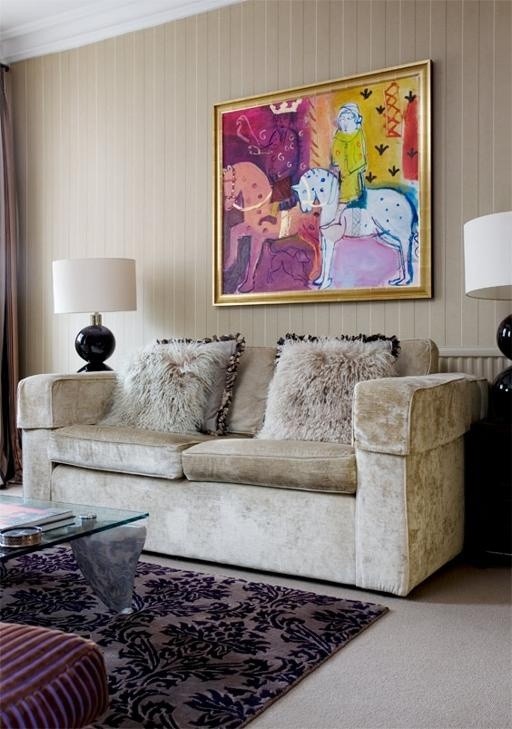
[461,207,512,421]
[50,254,138,372]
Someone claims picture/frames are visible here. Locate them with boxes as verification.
[209,56,437,308]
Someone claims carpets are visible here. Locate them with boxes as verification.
[0,543,389,729]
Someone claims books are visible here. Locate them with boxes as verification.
[0,501,73,532]
[1,516,76,538]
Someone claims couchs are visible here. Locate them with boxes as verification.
[14,336,493,601]
